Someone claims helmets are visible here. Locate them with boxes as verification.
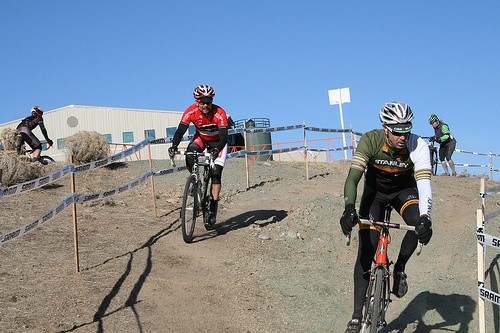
[193,84,215,100]
[379,102,414,124]
[429,115,437,123]
[31,107,43,116]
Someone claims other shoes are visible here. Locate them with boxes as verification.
[208,208,216,225]
[452,172,457,177]
[189,180,202,195]
[442,173,450,176]
[33,157,43,163]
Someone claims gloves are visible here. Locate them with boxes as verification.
[414,217,432,245]
[436,138,441,143]
[168,146,178,158]
[340,204,358,236]
[47,139,53,145]
[212,149,219,161]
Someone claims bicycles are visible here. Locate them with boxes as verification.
[345,209,424,333]
[428,136,438,176]
[170,149,218,242]
[16,135,55,164]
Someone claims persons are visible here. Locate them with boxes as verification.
[16,105,53,164]
[168,86,228,225]
[340,102,432,333]
[428,114,457,177]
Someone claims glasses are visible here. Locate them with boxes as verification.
[195,97,214,105]
[385,126,410,137]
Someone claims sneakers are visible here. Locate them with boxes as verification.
[345,315,363,333]
[393,268,408,297]
